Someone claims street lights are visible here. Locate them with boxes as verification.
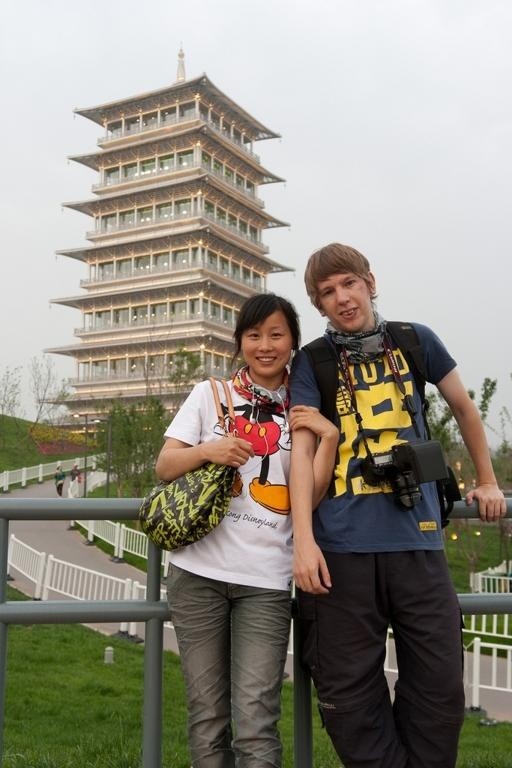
[90,416,113,501]
[69,413,89,503]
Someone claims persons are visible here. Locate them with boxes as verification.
[54,463,66,497]
[69,464,81,498]
[154,293,341,767]
[286,242,507,768]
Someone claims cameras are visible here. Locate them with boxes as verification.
[360,441,448,511]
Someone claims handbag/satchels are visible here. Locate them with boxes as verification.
[139,430,240,551]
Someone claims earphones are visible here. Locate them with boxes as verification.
[250,413,256,425]
[285,422,290,433]
